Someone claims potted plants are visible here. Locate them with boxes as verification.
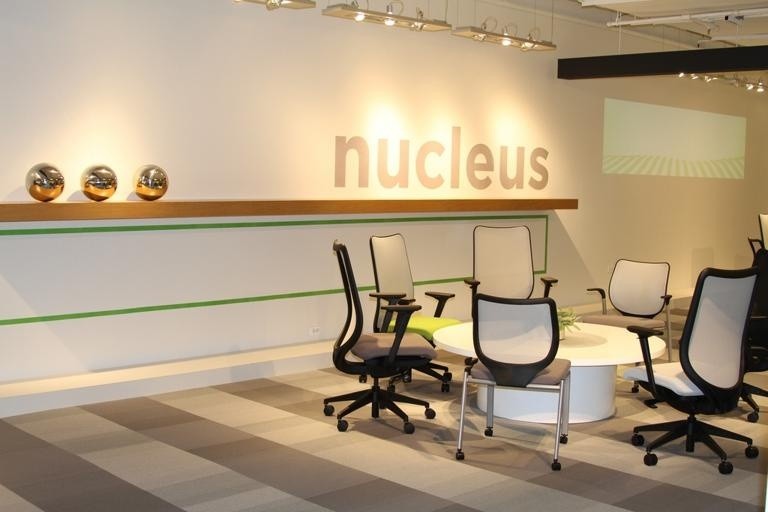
[555,308,580,340]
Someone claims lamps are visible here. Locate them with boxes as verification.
[324,1,453,32]
[255,1,316,10]
[451,2,556,52]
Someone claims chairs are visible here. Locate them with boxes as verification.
[621,248,765,474]
[322,239,437,435]
[716,248,765,424]
[464,224,559,302]
[371,233,456,392]
[456,293,572,471]
[582,258,672,394]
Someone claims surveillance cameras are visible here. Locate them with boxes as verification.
[724,15,739,25]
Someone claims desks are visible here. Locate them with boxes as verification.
[432,320,666,424]
